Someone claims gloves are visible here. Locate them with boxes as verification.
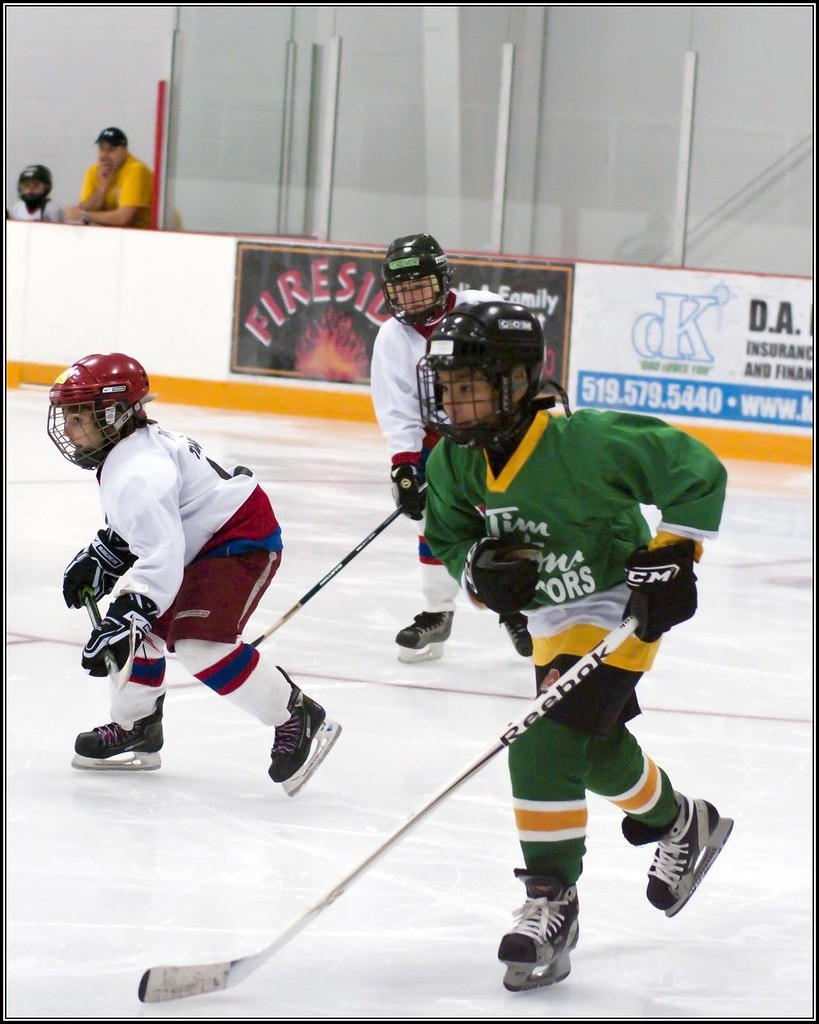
[465,536,544,616]
[80,594,158,677]
[621,539,698,644]
[391,462,427,520]
[63,529,139,609]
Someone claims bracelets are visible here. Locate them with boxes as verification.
[82,209,91,224]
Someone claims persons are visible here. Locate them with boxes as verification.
[64,127,181,231]
[416,300,734,991]
[46,352,343,797]
[8,165,64,222]
[370,233,506,665]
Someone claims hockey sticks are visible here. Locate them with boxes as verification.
[248,482,436,653]
[79,591,140,690]
[137,614,642,1004]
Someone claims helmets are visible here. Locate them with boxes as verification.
[17,165,52,201]
[416,300,572,449]
[47,353,155,469]
[380,232,456,326]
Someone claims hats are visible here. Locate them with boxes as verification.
[95,127,127,146]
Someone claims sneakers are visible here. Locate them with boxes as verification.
[268,666,343,797]
[71,691,165,771]
[498,857,583,992]
[395,611,454,664]
[499,612,533,657]
[622,790,734,918]
